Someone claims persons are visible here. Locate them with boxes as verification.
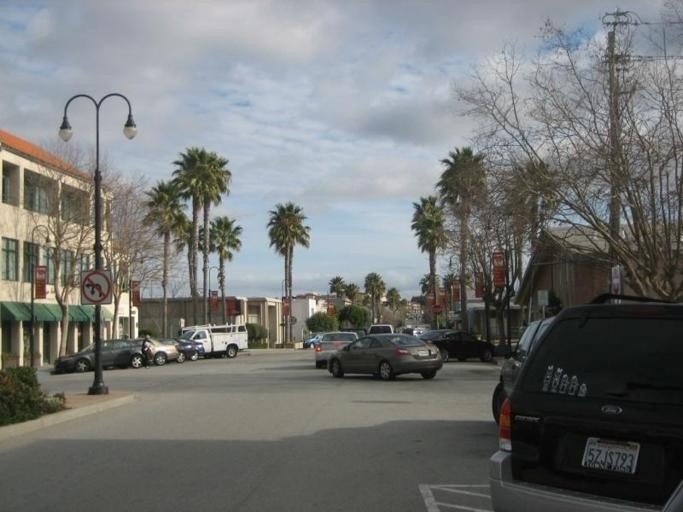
[141,334,155,369]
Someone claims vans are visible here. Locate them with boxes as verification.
[179,324,248,358]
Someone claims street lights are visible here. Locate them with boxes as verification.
[59,94,136,397]
[29,225,51,365]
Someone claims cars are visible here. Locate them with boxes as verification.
[368,325,394,334]
[327,333,443,381]
[133,338,204,367]
[54,339,137,374]
[303,334,321,349]
[315,332,359,369]
[419,329,495,362]
